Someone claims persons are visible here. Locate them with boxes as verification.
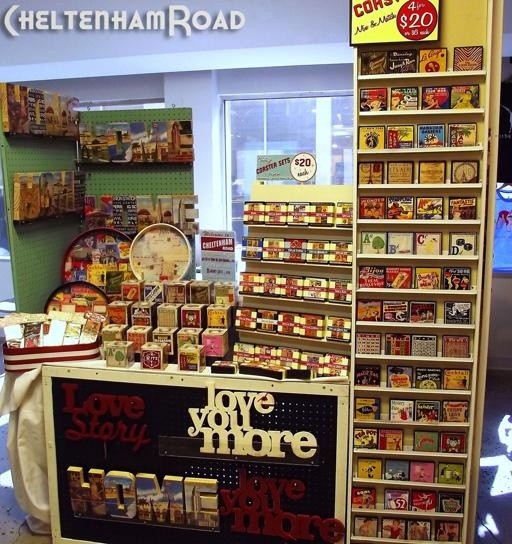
[364,463,376,478]
[409,522,430,540]
[383,520,404,540]
[361,493,374,509]
[393,437,401,450]
[358,518,375,537]
[461,275,469,289]
[439,529,446,540]
[446,435,461,453]
[366,436,375,446]
[418,310,427,321]
[426,309,433,320]
[419,468,425,482]
[355,432,366,444]
[359,86,476,112]
[445,275,454,289]
[446,524,458,541]
[452,275,461,290]
[418,492,434,511]
